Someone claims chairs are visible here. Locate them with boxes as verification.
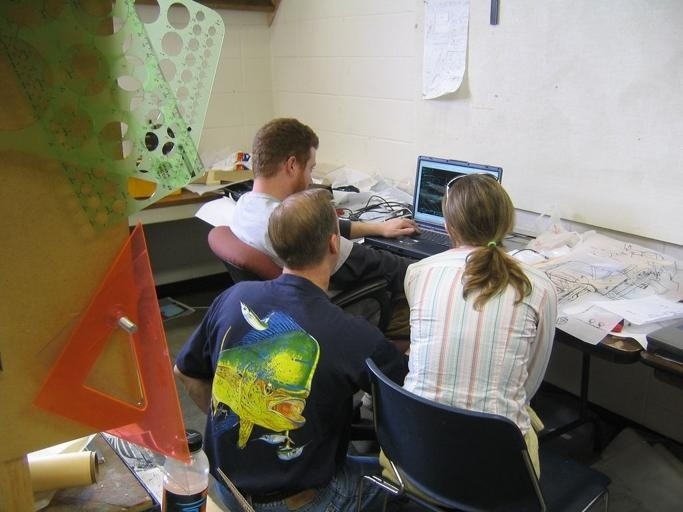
[359,359,612,512]
[208,226,394,334]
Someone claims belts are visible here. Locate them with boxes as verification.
[241,492,285,505]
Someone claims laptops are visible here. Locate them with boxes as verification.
[365,155,502,259]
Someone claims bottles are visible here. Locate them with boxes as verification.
[236,150,250,171]
[159,429,208,511]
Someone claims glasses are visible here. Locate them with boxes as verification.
[444,172,501,201]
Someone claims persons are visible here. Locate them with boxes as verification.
[227,116,422,328]
[169,187,410,512]
[378,173,559,507]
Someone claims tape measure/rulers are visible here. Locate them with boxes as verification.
[0,0,226,235]
[32,219,192,465]
